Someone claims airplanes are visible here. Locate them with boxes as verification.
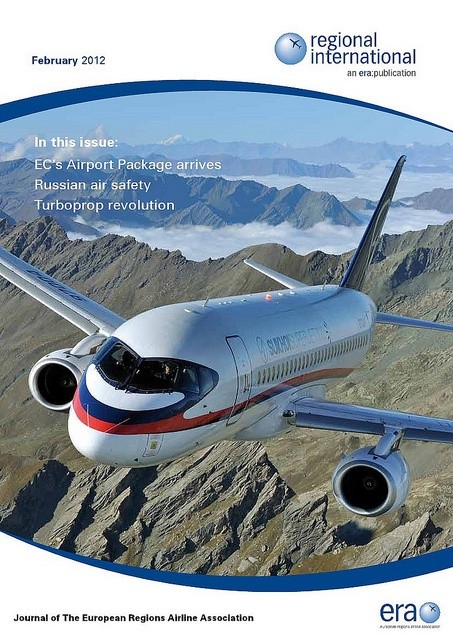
[0,155,453,517]
